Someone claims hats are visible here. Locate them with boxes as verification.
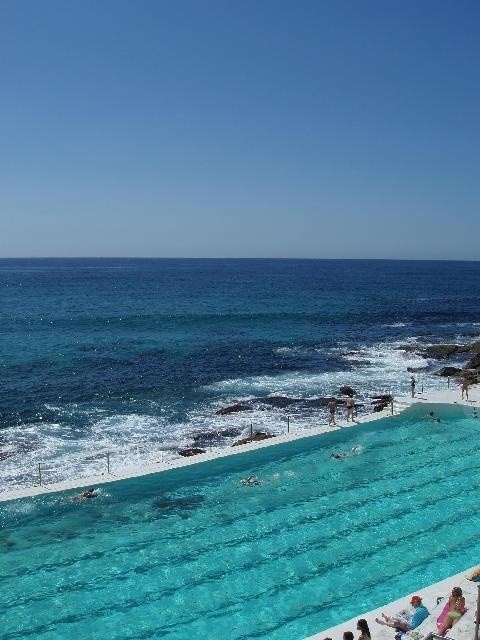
[409,596,422,604]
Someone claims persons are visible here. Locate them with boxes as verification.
[346,394,354,422]
[326,397,337,426]
[437,587,465,636]
[457,377,473,400]
[343,631,354,640]
[375,596,429,631]
[357,619,371,640]
